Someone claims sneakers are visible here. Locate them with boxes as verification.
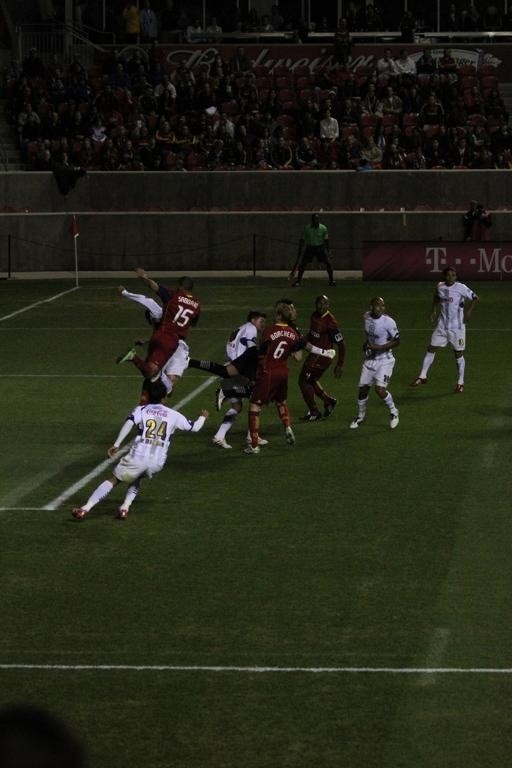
[410,377,427,386]
[350,415,367,430]
[71,508,88,520]
[117,348,136,366]
[389,408,400,429]
[298,411,322,422]
[292,280,304,287]
[323,398,337,418]
[284,425,295,446]
[118,508,128,520]
[328,281,336,288]
[454,384,464,394]
[215,387,226,410]
[212,435,268,455]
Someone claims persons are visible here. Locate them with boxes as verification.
[70,381,210,519]
[113,268,345,452]
[472,204,492,241]
[410,266,480,394]
[3,0,512,170]
[288,213,337,287]
[462,200,483,241]
[348,295,401,431]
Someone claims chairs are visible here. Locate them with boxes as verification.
[1,49,512,225]
